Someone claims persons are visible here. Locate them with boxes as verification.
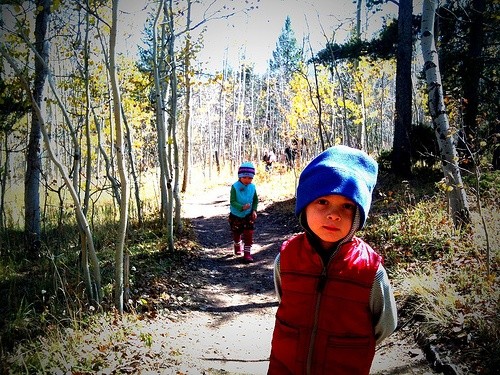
[228,161,259,263]
[266,144,398,375]
[263,138,309,171]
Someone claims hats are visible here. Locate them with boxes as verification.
[238,162,255,179]
[295,145,378,233]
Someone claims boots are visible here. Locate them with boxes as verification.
[244,245,253,262]
[234,243,242,255]
